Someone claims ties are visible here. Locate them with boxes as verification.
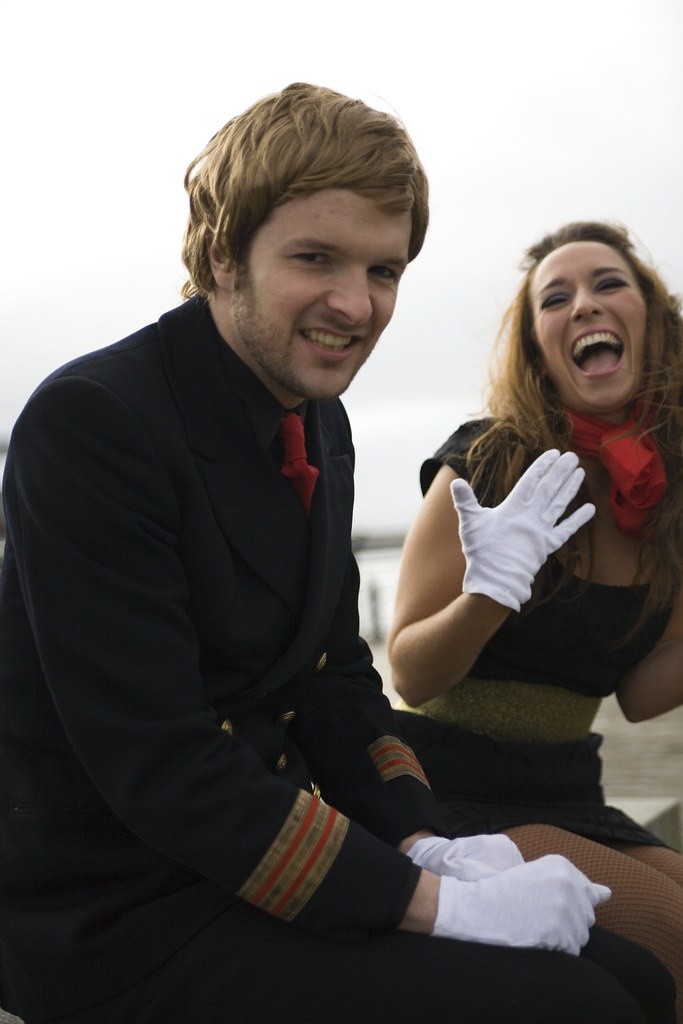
[280,412,318,515]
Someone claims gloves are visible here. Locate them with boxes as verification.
[450,449,595,613]
[406,834,525,883]
[431,854,611,956]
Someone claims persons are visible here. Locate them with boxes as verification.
[390,217,682,987]
[0,83,676,1024]
